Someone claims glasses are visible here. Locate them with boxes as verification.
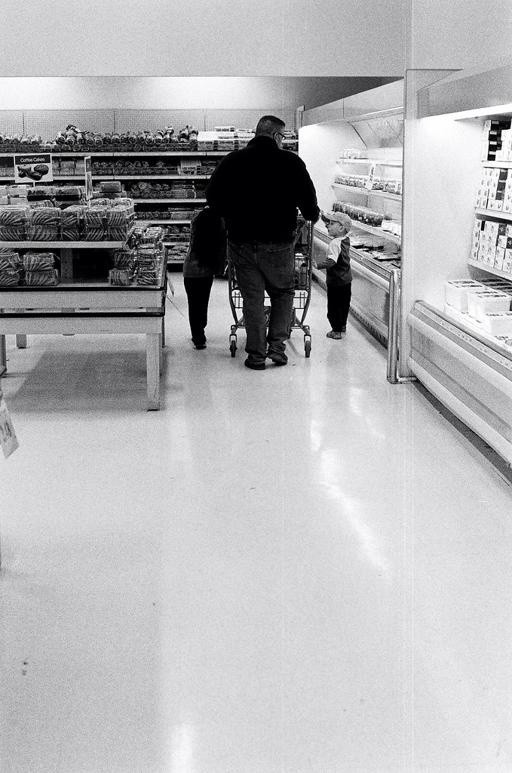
[274,131,286,140]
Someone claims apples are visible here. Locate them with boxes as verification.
[18,164,48,181]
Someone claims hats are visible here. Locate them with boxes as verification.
[322,213,351,233]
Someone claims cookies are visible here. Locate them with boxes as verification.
[0,199,162,286]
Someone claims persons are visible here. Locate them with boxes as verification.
[310,209,355,338]
[181,204,226,351]
[206,112,322,371]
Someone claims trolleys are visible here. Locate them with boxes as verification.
[225,215,313,357]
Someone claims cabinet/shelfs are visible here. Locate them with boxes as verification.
[0,136,297,267]
[0,240,167,410]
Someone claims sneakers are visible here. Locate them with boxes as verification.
[267,349,288,365]
[341,325,346,332]
[196,340,208,350]
[245,359,265,370]
[327,330,341,339]
